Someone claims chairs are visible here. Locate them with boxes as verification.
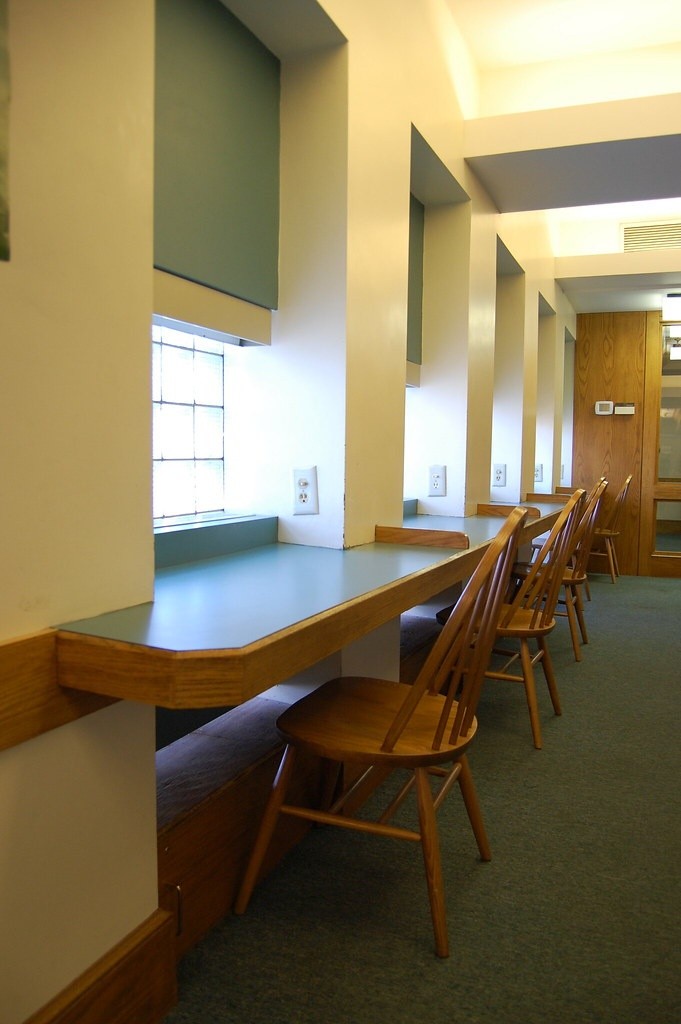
[232,475,635,957]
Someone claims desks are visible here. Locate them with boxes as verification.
[54,487,582,710]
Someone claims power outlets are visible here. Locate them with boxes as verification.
[534,464,543,482]
[491,464,506,487]
[294,467,318,515]
[428,465,446,496]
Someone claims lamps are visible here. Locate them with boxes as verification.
[670,326,681,360]
